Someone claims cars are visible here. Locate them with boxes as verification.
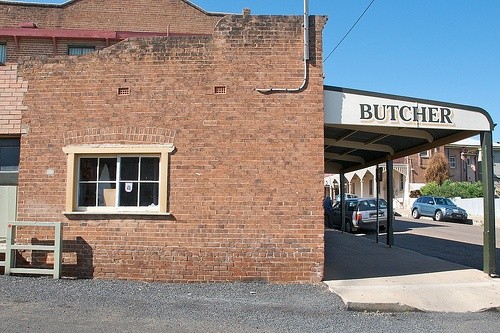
[325,197,395,231]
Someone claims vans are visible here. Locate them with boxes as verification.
[337,193,358,201]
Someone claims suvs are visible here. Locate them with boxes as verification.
[412,195,468,222]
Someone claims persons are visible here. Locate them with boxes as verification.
[324,196,334,228]
